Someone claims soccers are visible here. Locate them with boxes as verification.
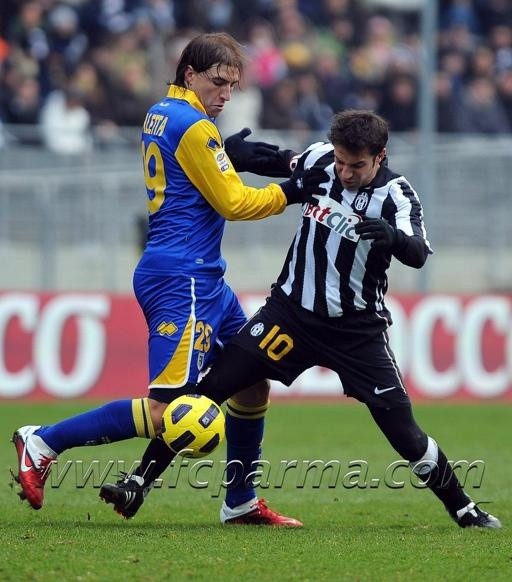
[162,395,226,459]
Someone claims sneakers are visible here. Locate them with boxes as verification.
[97,474,146,520]
[454,500,502,531]
[9,424,59,509]
[218,496,304,527]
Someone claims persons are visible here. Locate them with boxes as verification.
[10,31,333,532]
[0,0,512,139]
[94,109,504,534]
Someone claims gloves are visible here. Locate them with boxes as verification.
[353,215,399,253]
[222,126,280,175]
[278,160,327,207]
